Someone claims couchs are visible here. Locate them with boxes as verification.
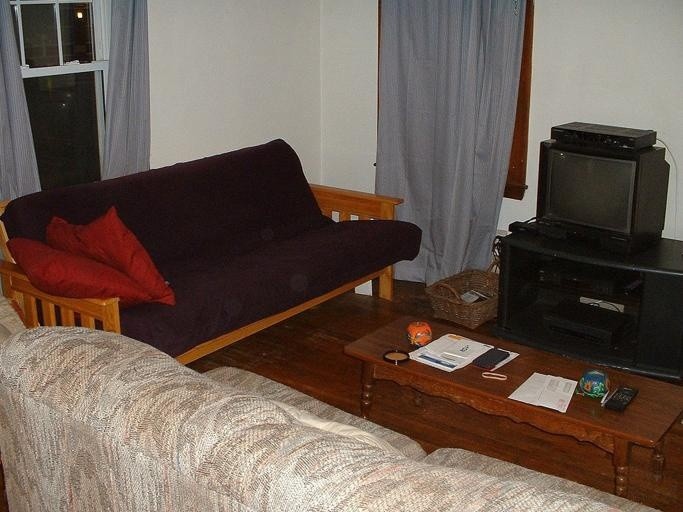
[0,323,682,511]
[0,138,423,369]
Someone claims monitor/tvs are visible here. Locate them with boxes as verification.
[536,137,671,255]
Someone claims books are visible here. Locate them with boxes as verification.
[405,332,522,376]
[535,374,578,414]
[506,371,562,407]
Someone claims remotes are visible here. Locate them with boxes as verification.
[604,383,639,413]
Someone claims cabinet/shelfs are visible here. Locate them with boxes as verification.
[487,220,683,385]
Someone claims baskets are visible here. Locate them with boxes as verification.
[424,261,500,330]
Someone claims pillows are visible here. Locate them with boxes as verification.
[4,206,177,308]
